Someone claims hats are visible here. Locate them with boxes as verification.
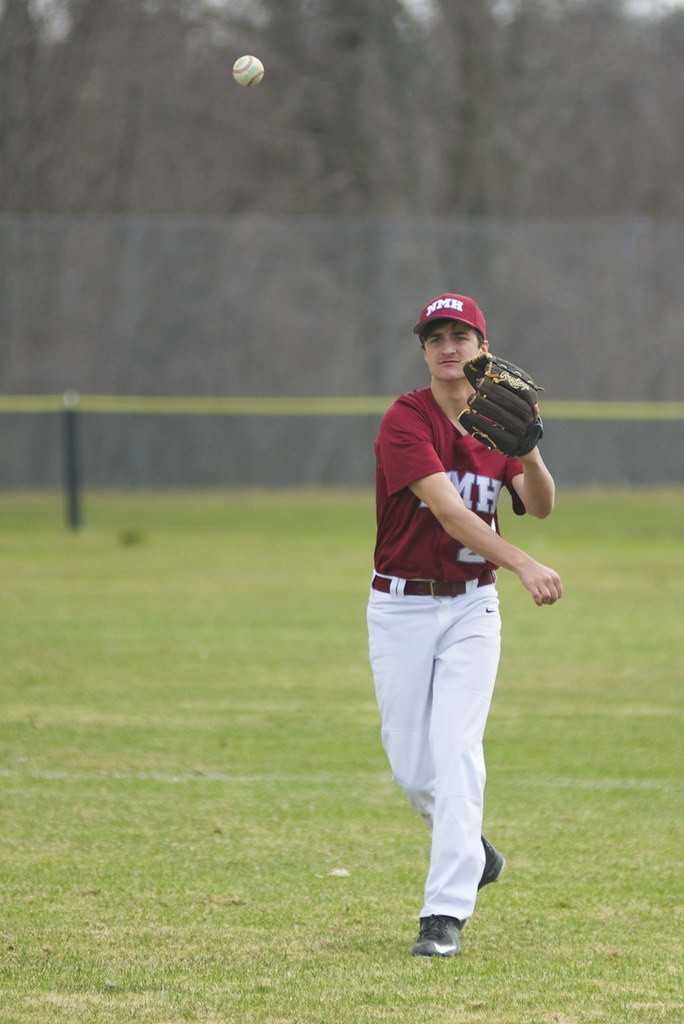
[414,292,487,339]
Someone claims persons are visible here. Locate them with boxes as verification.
[367,293,564,951]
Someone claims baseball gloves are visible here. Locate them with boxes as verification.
[457,352,545,457]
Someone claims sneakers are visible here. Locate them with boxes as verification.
[478,835,504,891]
[410,914,468,956]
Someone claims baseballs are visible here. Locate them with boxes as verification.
[232,54,266,88]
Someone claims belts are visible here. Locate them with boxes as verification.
[373,576,497,596]
